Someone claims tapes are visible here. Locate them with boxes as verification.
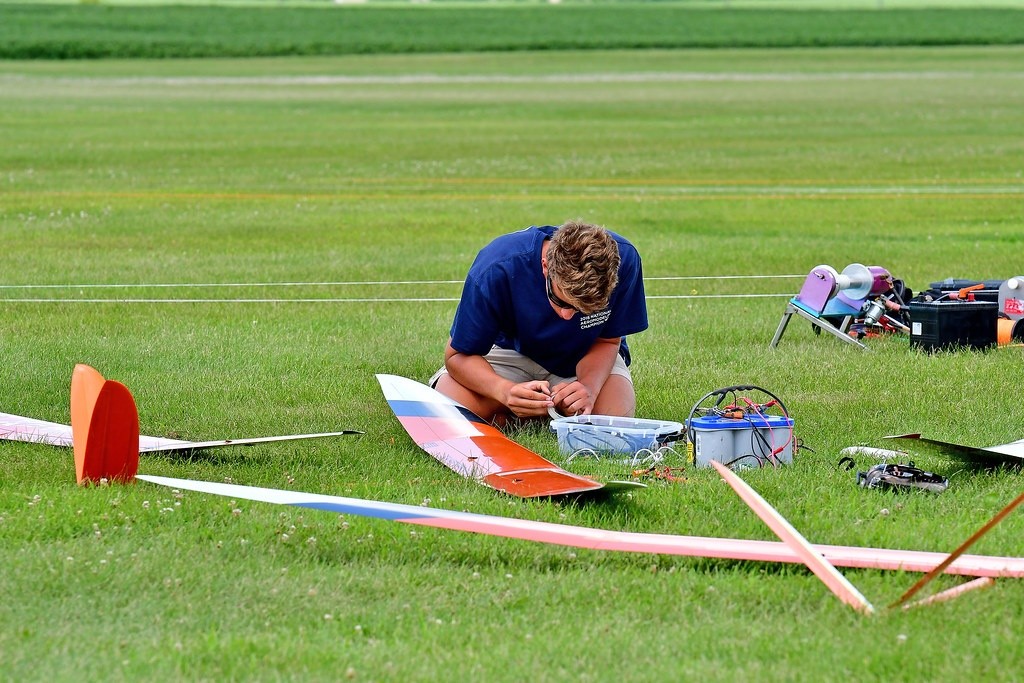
[546,395,579,420]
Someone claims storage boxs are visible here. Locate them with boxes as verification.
[550,414,683,458]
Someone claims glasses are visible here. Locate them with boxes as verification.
[547,274,581,313]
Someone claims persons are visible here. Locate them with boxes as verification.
[429,218,649,426]
